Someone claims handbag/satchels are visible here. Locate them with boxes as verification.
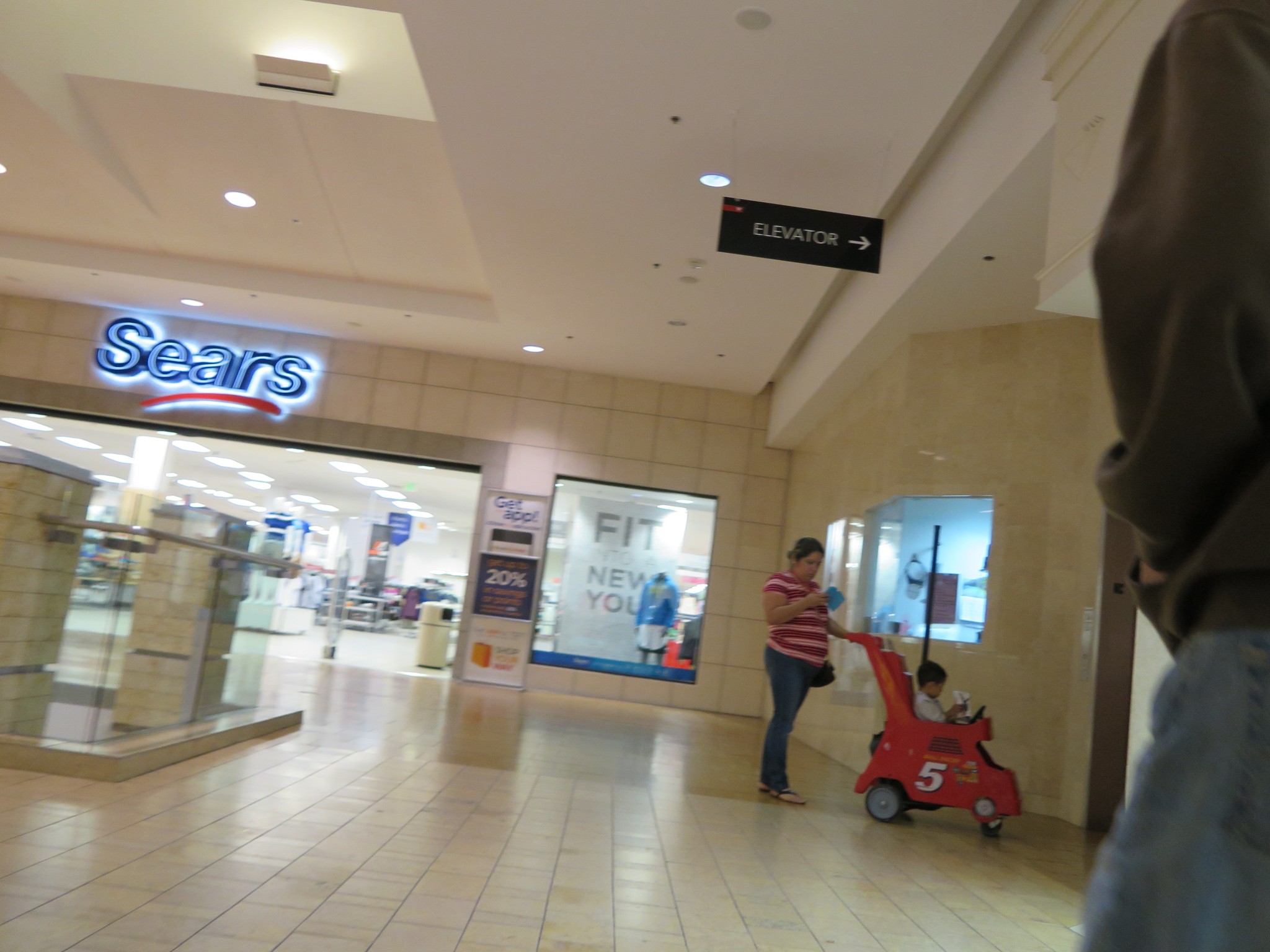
[809,659,835,688]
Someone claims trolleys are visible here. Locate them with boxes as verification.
[844,632,1026,838]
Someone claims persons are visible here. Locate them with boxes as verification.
[757,537,854,805]
[633,571,683,666]
[1077,0,1270,952]
[913,660,967,723]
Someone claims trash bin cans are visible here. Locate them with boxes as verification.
[414,601,454,669]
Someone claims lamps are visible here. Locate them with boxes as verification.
[253,54,341,98]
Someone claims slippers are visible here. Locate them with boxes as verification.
[770,790,804,803]
[759,788,771,792]
